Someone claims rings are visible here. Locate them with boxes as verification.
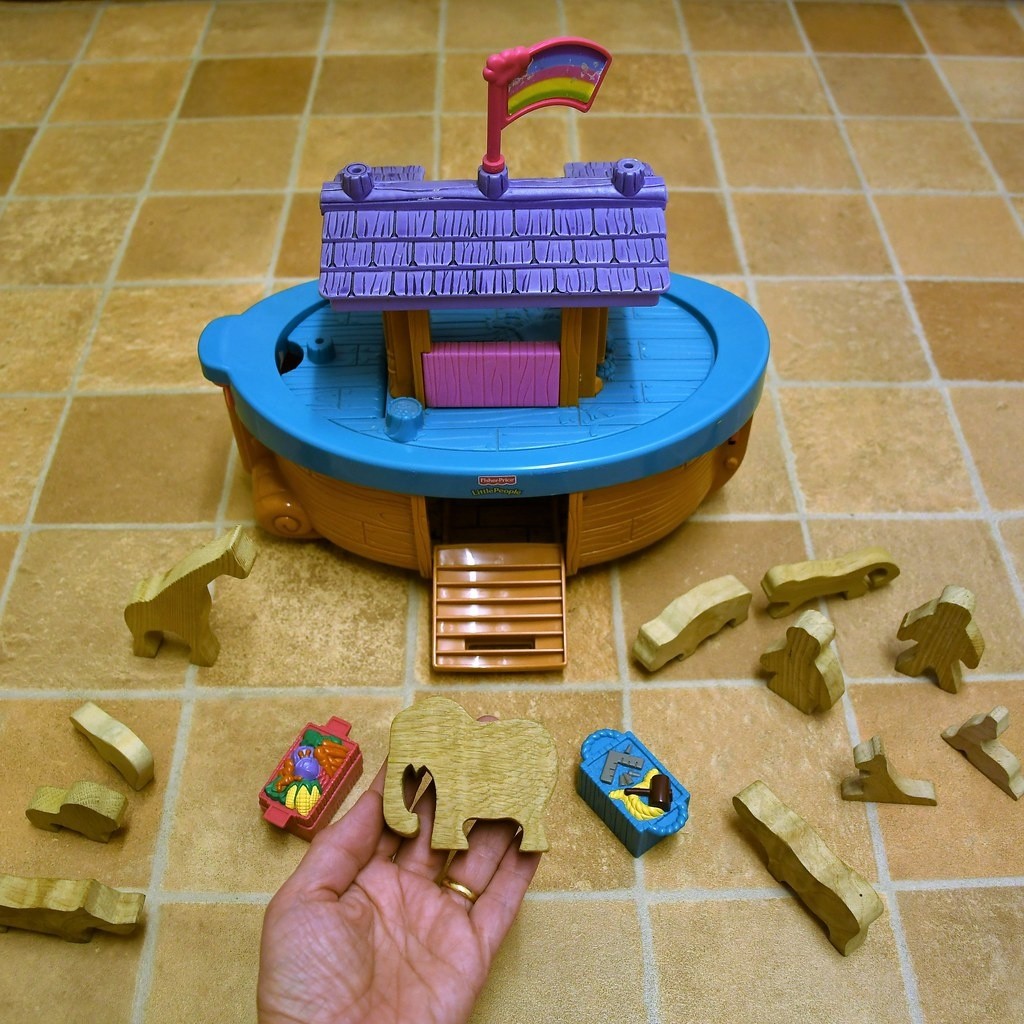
[440,877,479,905]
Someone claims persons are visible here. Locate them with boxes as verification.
[257,715,543,1024]
